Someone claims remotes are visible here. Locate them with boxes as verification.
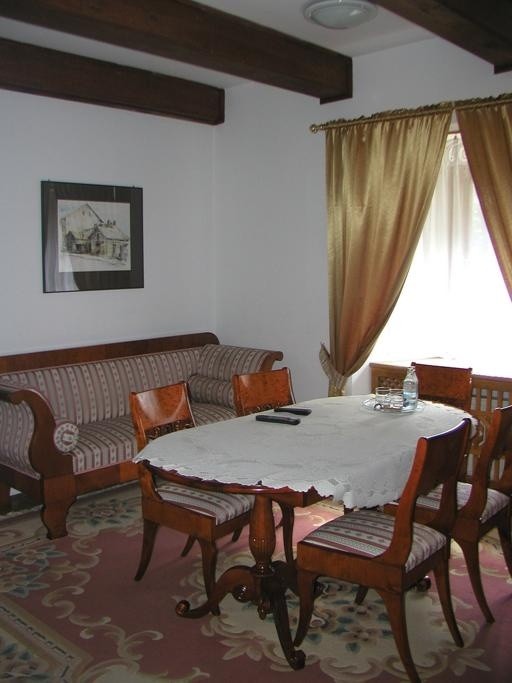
[273,405,311,416]
[254,413,301,426]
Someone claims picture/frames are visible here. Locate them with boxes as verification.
[40,180,144,295]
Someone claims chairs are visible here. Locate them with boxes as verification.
[128,380,294,616]
[291,417,472,682]
[230,367,296,529]
[413,361,473,407]
[373,405,511,625]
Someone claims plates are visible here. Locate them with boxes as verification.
[360,397,426,414]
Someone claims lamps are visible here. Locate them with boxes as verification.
[303,0,378,34]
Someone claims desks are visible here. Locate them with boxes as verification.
[129,393,478,671]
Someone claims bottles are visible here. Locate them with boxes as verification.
[403,361,419,410]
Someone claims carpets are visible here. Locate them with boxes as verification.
[0,478,512,683]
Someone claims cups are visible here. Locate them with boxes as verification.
[374,386,404,408]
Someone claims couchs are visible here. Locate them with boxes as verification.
[0,330,284,539]
